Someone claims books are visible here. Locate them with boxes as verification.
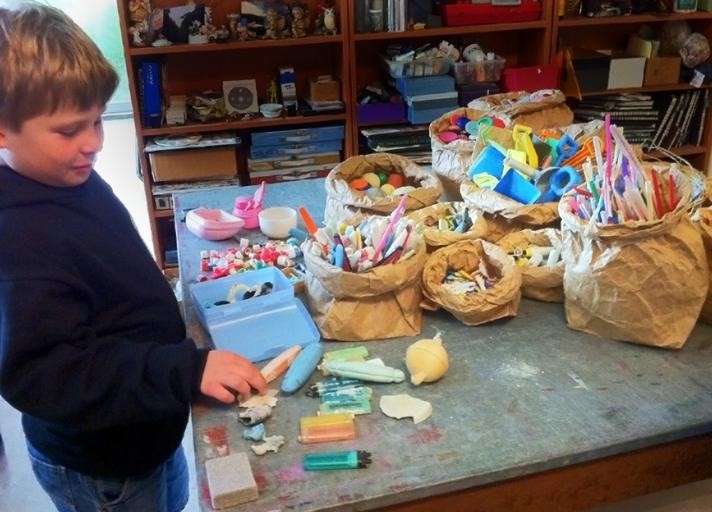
[355,0,408,34]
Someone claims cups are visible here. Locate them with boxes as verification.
[462,43,486,61]
[258,206,297,239]
[260,103,282,117]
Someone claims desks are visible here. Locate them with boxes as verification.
[167,166,711,509]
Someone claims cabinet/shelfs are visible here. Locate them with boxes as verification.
[117,0,349,280]
[350,0,711,175]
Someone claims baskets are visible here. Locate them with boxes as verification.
[459,86,502,106]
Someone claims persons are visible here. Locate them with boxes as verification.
[0,0,269,512]
